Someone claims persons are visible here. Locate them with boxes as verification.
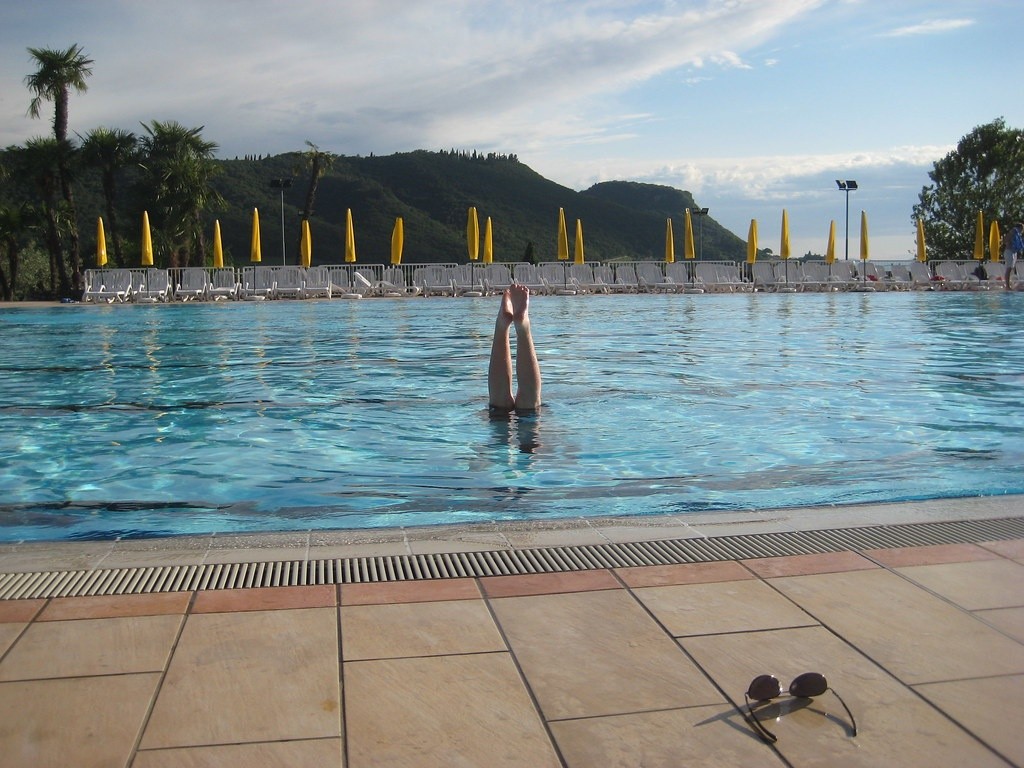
[1004,223,1024,290]
[488,284,541,408]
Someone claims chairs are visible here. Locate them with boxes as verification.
[82,261,1024,303]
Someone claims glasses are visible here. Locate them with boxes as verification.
[745,672,857,741]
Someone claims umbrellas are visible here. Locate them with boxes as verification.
[684,207,695,289]
[345,208,356,286]
[780,209,791,287]
[747,219,757,282]
[300,219,312,266]
[97,216,108,285]
[558,207,569,290]
[574,218,584,264]
[390,216,403,285]
[973,207,1000,284]
[860,211,868,287]
[142,210,154,297]
[466,206,492,292]
[826,220,835,281]
[665,218,675,264]
[213,219,223,287]
[917,219,926,263]
[250,207,261,294]
